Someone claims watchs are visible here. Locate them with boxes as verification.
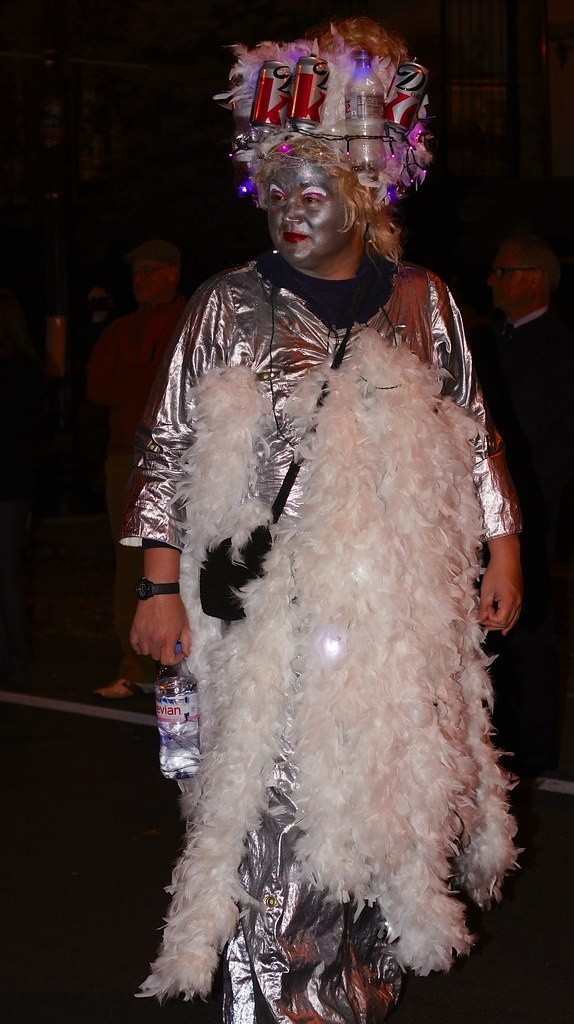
[136,577,180,600]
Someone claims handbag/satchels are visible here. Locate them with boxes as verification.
[199,525,270,622]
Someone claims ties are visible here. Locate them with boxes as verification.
[501,323,515,345]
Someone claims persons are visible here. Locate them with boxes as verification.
[467,232,573,779]
[119,20,526,1024]
[86,240,188,699]
[0,289,46,689]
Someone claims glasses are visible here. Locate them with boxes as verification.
[130,264,171,276]
[492,263,544,280]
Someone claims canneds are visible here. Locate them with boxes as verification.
[249,59,294,132]
[283,55,329,129]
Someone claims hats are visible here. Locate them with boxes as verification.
[124,240,181,265]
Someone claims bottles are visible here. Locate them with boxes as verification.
[155,642,200,779]
[345,50,386,170]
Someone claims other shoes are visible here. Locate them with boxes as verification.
[96,679,151,699]
